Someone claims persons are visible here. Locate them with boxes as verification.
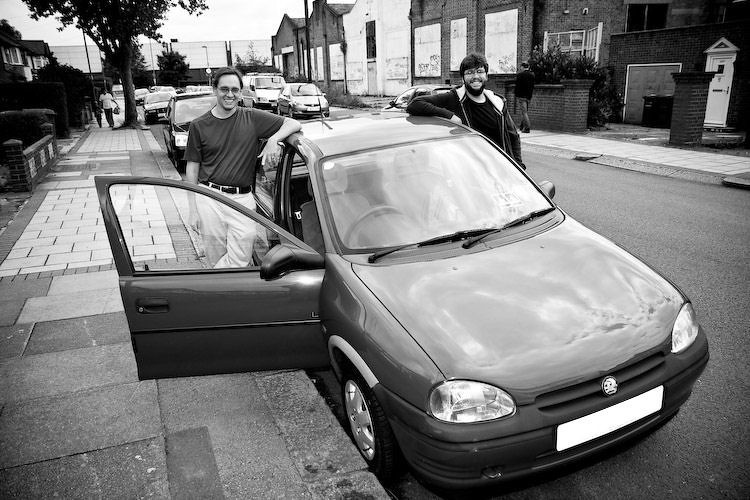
[182,67,302,269]
[406,53,527,172]
[93,88,118,129]
[515,63,534,133]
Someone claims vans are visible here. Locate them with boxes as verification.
[238,72,286,109]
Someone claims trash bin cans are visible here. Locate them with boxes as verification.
[642,95,673,128]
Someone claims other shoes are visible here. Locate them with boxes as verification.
[523,128,530,133]
[520,125,524,130]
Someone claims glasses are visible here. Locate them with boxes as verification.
[215,87,239,93]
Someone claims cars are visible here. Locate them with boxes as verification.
[277,83,330,119]
[140,92,174,118]
[382,83,459,112]
[94,111,709,500]
[163,91,218,173]
[134,85,184,106]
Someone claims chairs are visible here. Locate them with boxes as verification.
[175,104,191,124]
[383,145,467,236]
[298,160,373,253]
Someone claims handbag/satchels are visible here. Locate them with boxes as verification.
[114,106,120,114]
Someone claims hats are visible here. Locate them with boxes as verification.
[522,63,529,68]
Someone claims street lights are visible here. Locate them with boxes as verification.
[202,46,209,69]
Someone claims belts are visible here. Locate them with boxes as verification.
[201,181,252,194]
